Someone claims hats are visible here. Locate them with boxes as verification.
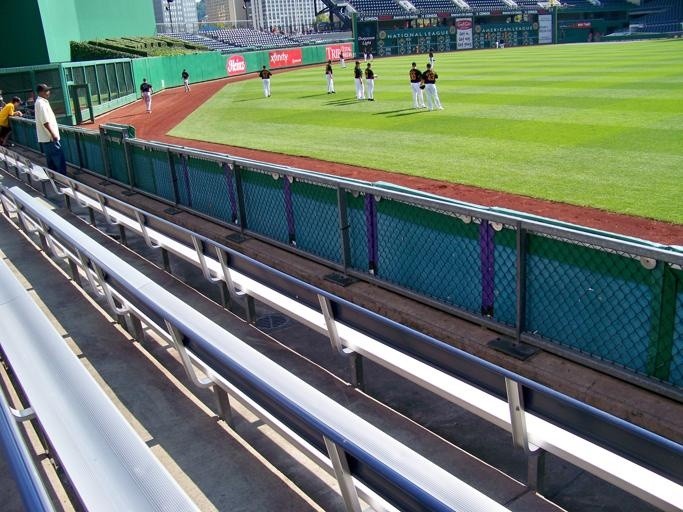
[12,97,24,104]
[37,84,53,91]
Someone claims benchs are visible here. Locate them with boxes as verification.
[0,143,682,511]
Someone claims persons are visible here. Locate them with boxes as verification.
[428,48,435,71]
[140,77,154,114]
[421,64,443,111]
[31,83,67,176]
[368,46,374,62]
[409,62,427,109]
[325,59,335,94]
[353,61,364,100]
[180,69,191,93]
[365,63,375,101]
[0,95,22,151]
[338,51,346,68]
[0,92,35,119]
[258,65,272,98]
[362,46,367,63]
